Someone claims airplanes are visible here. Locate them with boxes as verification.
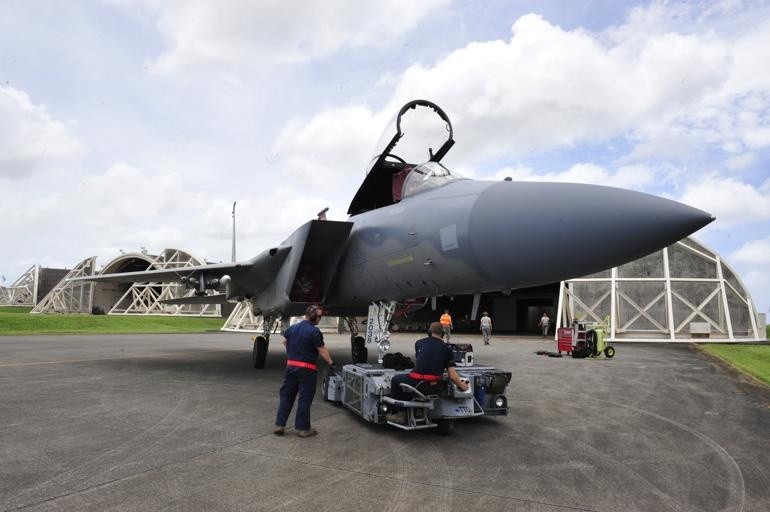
[64,94,718,370]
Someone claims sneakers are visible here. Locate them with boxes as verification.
[386,412,406,424]
[485,341,489,345]
[274,426,284,435]
[298,428,317,438]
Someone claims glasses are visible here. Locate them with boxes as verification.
[316,315,321,318]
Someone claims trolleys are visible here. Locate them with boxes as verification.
[584,315,615,360]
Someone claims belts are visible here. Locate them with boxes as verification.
[409,372,441,380]
[287,359,317,371]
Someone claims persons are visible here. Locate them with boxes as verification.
[385,322,470,425]
[439,309,453,343]
[274,304,336,436]
[538,313,550,336]
[480,311,492,345]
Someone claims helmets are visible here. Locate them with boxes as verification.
[428,322,443,333]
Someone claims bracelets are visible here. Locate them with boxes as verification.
[329,361,335,369]
